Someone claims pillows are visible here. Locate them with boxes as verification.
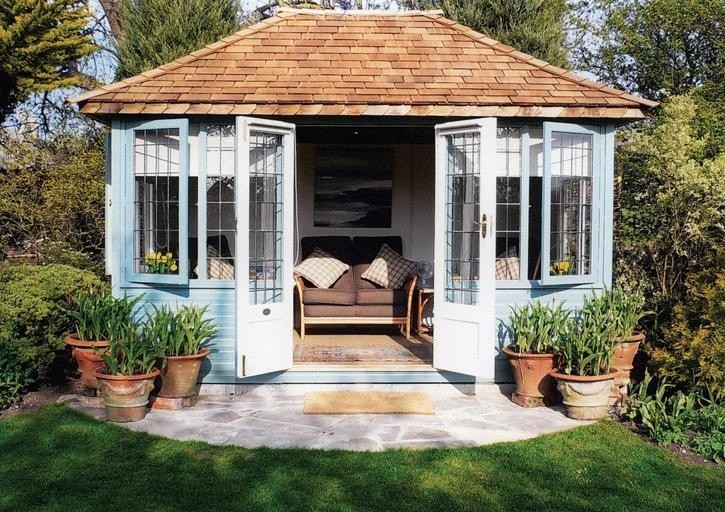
[495,244,520,281]
[192,244,236,281]
[295,243,419,290]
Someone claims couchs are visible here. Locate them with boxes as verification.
[293,235,418,340]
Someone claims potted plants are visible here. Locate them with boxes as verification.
[58,285,219,423]
[496,284,658,421]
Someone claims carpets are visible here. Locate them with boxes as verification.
[302,390,437,416]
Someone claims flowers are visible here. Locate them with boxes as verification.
[549,259,575,275]
[145,251,178,275]
